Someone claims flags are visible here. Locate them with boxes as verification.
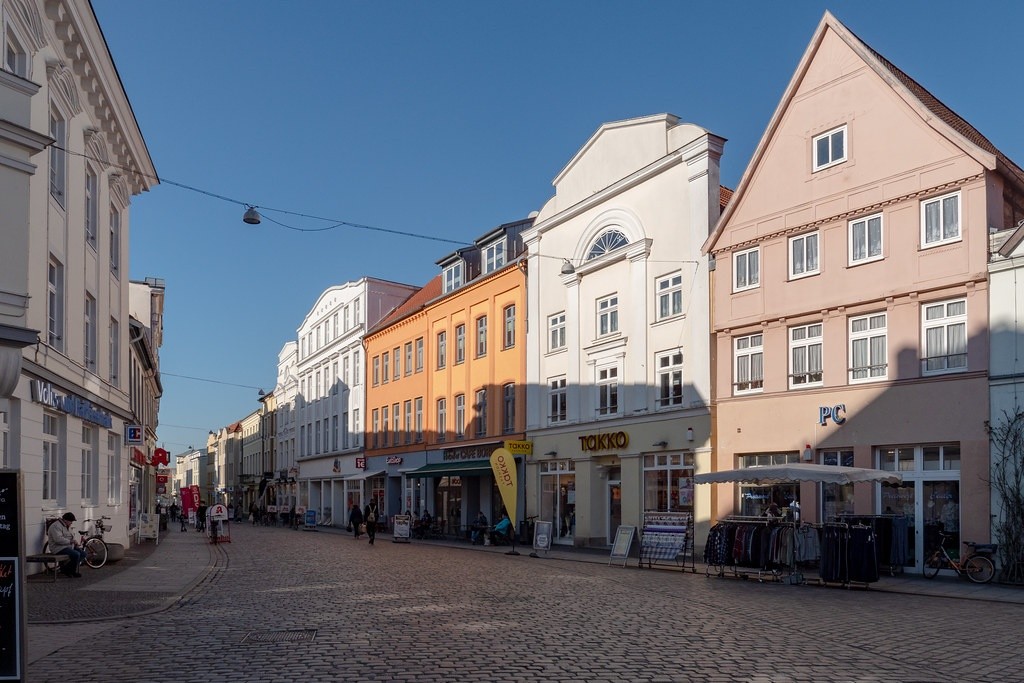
[490,447,518,531]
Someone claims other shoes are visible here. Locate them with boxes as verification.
[369,538,374,544]
[357,536,360,540]
[353,537,356,539]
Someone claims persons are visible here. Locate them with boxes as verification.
[471,512,487,542]
[249,503,259,524]
[196,500,208,530]
[492,513,511,545]
[169,503,178,522]
[48,512,87,578]
[364,498,379,545]
[349,505,363,539]
[405,509,431,537]
[228,502,243,522]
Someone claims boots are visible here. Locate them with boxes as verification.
[61,562,82,578]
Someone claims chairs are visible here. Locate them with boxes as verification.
[432,520,447,539]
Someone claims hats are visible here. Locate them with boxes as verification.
[62,512,77,521]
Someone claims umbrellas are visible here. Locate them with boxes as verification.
[692,462,902,574]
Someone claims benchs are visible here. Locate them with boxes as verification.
[26,553,73,583]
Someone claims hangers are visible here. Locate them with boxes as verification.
[712,516,907,539]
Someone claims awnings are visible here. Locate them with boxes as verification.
[405,458,519,479]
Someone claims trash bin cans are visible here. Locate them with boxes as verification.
[519,521,529,542]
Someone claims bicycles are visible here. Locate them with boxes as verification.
[41,515,109,572]
[84,515,112,556]
[923,530,996,585]
[293,514,302,530]
[526,515,539,544]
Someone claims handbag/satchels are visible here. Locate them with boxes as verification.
[358,524,366,534]
[346,525,352,532]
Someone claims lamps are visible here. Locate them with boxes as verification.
[258,389,265,395]
[209,431,213,434]
[242,207,261,224]
[561,259,575,274]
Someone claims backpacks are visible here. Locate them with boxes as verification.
[367,505,377,523]
